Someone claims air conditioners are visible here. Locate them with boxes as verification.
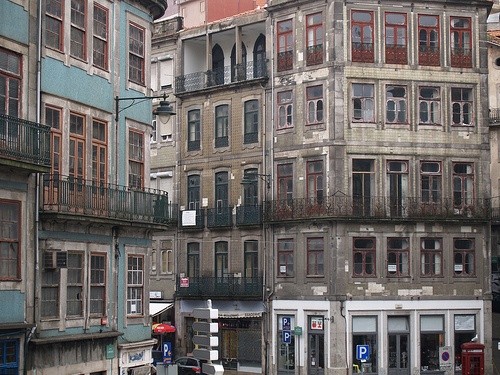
[42,250,68,268]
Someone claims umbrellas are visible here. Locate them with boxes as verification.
[151,321,176,352]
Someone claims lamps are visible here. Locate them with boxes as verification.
[240,171,271,190]
[115,92,177,125]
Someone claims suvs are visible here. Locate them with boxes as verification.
[172,357,208,375]
